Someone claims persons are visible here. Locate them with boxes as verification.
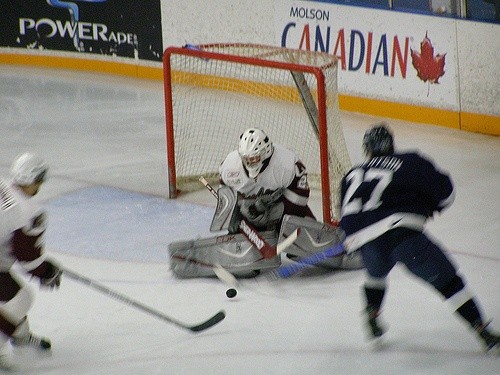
[217,128,317,278]
[1,152,62,371]
[339,125,500,353]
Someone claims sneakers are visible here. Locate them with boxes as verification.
[367,321,387,351]
[10,333,51,356]
[0,352,17,372]
[470,322,500,354]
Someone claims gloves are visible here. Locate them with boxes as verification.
[41,266,62,288]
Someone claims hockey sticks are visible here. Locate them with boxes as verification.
[198,176,302,259]
[214,243,346,290]
[61,269,226,331]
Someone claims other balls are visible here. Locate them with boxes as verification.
[226,288,237,298]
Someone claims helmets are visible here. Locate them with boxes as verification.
[238,127,272,179]
[10,152,49,187]
